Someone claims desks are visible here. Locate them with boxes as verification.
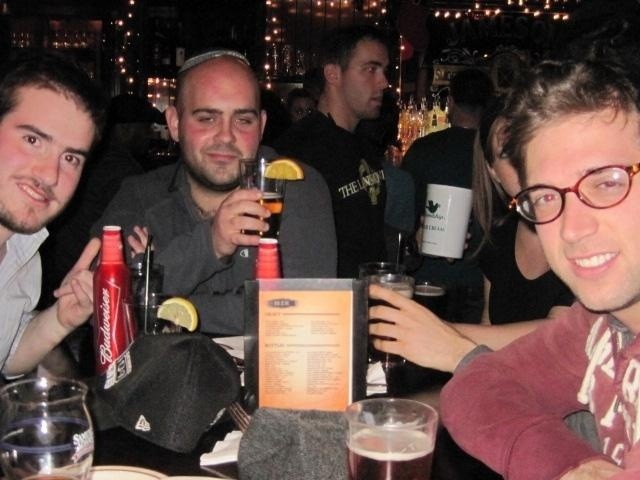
[0,334,503,480]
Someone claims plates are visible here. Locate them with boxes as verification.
[90,465,168,480]
[211,334,245,369]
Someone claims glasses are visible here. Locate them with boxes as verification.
[507,162,640,224]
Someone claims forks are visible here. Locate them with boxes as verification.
[226,401,251,433]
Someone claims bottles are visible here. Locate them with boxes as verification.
[388,94,450,167]
[93,226,136,375]
[256,239,283,281]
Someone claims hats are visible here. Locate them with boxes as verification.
[80,334,244,453]
[178,50,251,74]
[450,68,483,105]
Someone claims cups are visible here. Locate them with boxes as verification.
[345,398,439,480]
[128,260,164,330]
[358,262,415,368]
[0,376,95,480]
[239,158,285,238]
[420,184,473,260]
[413,281,447,317]
[151,315,182,335]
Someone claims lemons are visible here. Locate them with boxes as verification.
[157,298,198,333]
[265,159,304,182]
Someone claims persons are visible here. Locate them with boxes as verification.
[0,27,640,480]
